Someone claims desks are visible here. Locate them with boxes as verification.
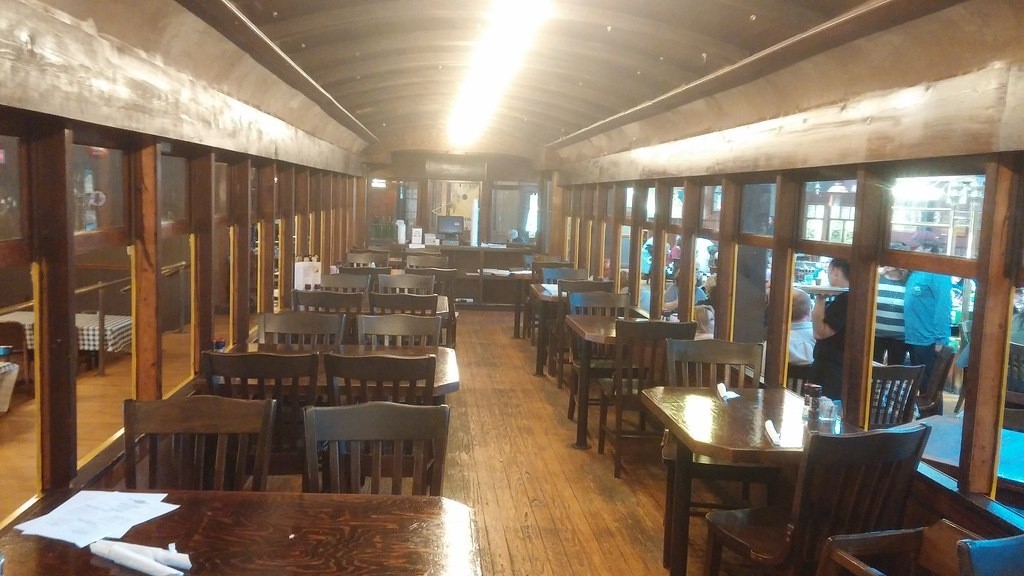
[0,311,132,369]
[639,386,867,576]
[219,344,460,486]
[300,295,449,344]
[0,491,485,576]
[890,415,1024,494]
[564,314,665,450]
[529,283,568,379]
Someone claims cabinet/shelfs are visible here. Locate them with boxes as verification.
[442,246,538,310]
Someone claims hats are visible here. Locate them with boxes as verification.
[902,231,938,248]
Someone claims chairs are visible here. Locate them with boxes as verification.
[523,259,1024,576]
[124,237,458,496]
[868,364,929,430]
[0,321,34,388]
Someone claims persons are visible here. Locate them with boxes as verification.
[602,213,1024,421]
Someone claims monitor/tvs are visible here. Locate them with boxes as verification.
[437,215,464,235]
